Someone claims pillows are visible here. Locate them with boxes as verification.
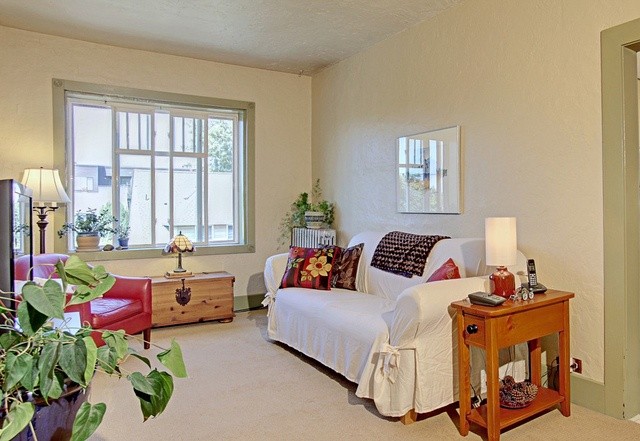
[278,241,339,293]
[319,243,363,292]
[422,258,461,284]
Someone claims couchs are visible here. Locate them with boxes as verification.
[11,254,152,349]
[266,231,530,431]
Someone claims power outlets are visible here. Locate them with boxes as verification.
[572,357,583,375]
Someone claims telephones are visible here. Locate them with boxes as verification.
[469,291,505,307]
[520,259,548,294]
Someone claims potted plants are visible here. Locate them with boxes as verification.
[1,256,189,441]
[276,178,337,251]
[115,226,132,248]
[57,208,117,254]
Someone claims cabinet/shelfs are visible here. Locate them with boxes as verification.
[144,272,236,328]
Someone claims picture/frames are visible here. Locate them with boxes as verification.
[394,126,463,214]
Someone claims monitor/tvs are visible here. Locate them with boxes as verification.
[0,179,33,324]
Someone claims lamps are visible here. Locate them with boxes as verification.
[19,169,72,252]
[164,230,197,279]
[483,217,519,296]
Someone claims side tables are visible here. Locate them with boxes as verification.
[454,284,575,441]
